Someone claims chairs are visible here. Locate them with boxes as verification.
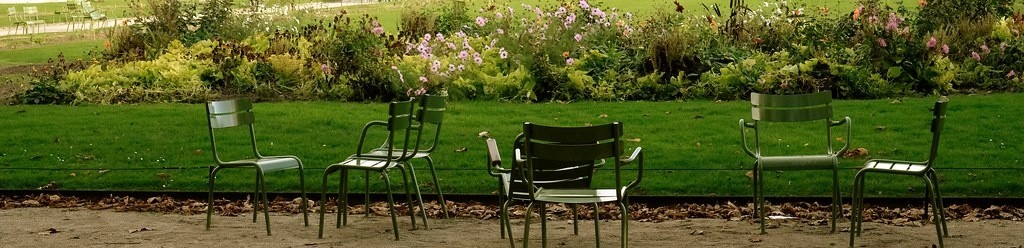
[205,97,308,235]
[343,90,450,230]
[318,97,417,241]
[739,90,852,234]
[849,95,949,248]
[515,121,643,248]
[486,137,606,248]
